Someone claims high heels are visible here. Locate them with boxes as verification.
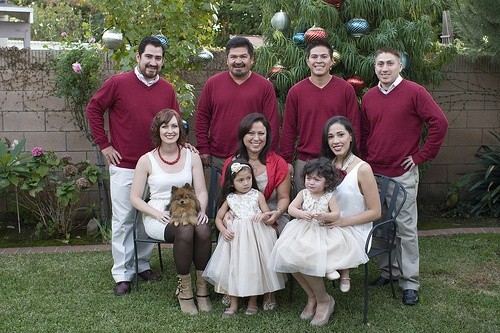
[302,305,316,321]
[310,294,336,326]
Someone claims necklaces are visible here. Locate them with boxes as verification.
[157,145,181,165]
[332,155,357,171]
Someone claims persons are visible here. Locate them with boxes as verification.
[279,41,362,194]
[218,110,291,310]
[199,156,290,316]
[85,37,201,295]
[126,108,214,316]
[262,156,346,327]
[192,36,280,241]
[358,47,449,305]
[306,114,382,292]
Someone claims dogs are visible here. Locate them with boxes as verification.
[164,183,201,227]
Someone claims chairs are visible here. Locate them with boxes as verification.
[133,182,164,292]
[290,173,407,326]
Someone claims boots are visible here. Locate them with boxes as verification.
[175,274,198,316]
[194,270,213,312]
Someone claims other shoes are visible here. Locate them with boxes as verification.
[263,300,276,311]
[326,270,341,280]
[221,296,230,306]
[340,278,350,292]
[245,305,258,315]
[223,307,238,317]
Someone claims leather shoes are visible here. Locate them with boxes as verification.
[138,270,162,281]
[115,282,131,295]
[402,289,419,304]
[371,275,398,285]
